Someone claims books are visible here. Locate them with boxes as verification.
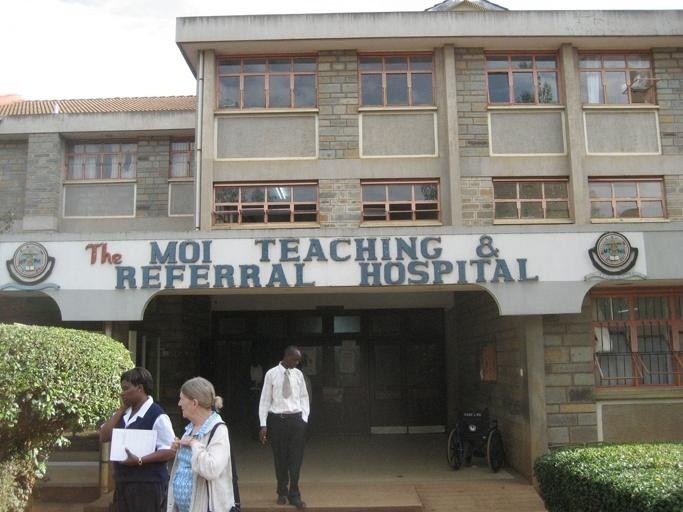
[110,428,158,461]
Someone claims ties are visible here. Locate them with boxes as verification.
[281,371,292,398]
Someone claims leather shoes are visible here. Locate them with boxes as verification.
[277,493,306,507]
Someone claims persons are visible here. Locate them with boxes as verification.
[258,346,312,507]
[165,376,235,512]
[97,367,176,511]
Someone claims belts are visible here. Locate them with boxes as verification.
[272,414,302,419]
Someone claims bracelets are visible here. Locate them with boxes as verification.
[185,436,193,447]
[138,456,144,467]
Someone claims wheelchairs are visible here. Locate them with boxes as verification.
[448,407,504,474]
